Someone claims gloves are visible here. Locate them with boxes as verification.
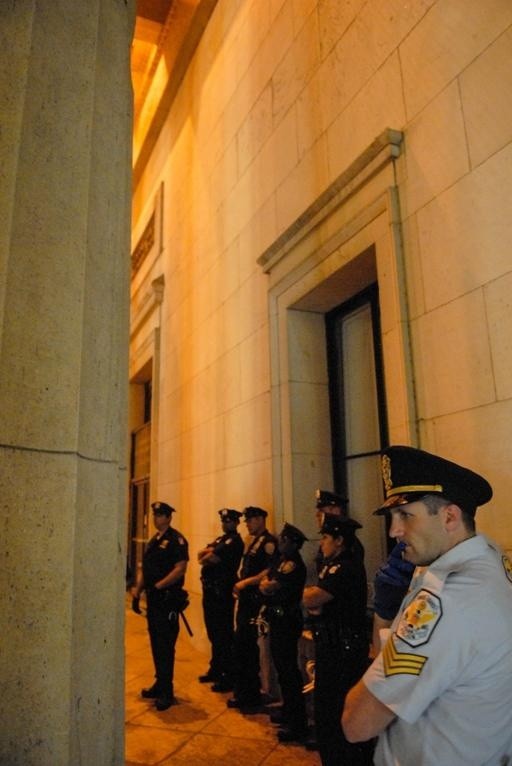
[132,597,142,615]
[373,542,413,618]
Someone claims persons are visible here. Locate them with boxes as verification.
[301,490,368,766]
[340,446,512,766]
[198,507,307,740]
[132,501,190,709]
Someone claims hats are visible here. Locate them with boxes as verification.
[316,489,349,508]
[218,509,242,523]
[372,445,492,515]
[319,513,362,534]
[278,522,309,543]
[151,502,175,514]
[243,507,268,521]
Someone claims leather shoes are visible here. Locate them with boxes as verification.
[142,679,173,710]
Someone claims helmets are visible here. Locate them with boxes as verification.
[198,674,261,707]
